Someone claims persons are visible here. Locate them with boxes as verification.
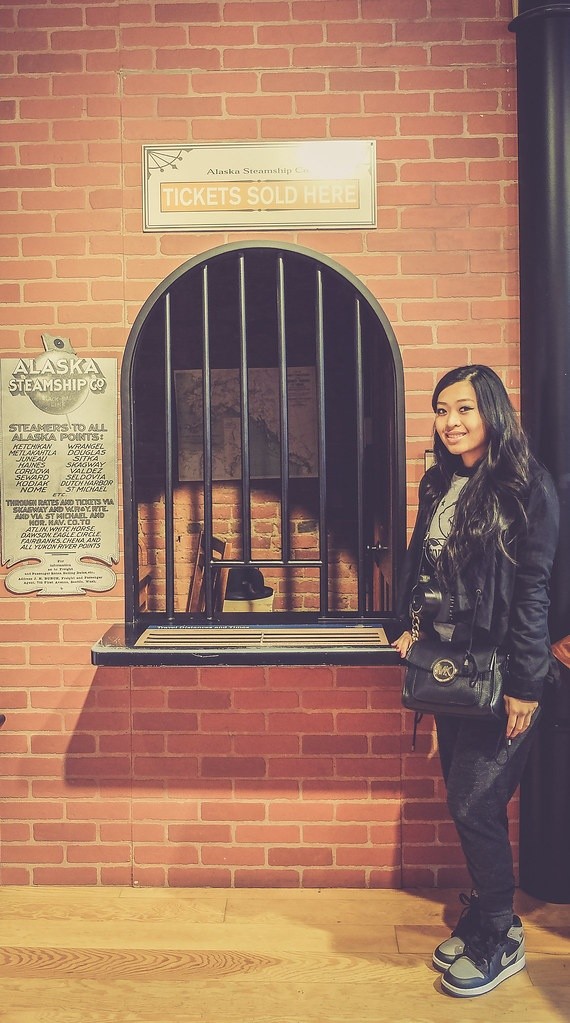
[391,364,561,997]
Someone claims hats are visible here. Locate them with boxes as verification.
[224,568,275,601]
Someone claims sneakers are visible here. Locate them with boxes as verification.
[440,914,526,998]
[432,890,513,973]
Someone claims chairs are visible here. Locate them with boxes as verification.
[186,530,232,612]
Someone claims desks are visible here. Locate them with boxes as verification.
[139,565,155,611]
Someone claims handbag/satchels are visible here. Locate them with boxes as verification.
[400,638,511,721]
